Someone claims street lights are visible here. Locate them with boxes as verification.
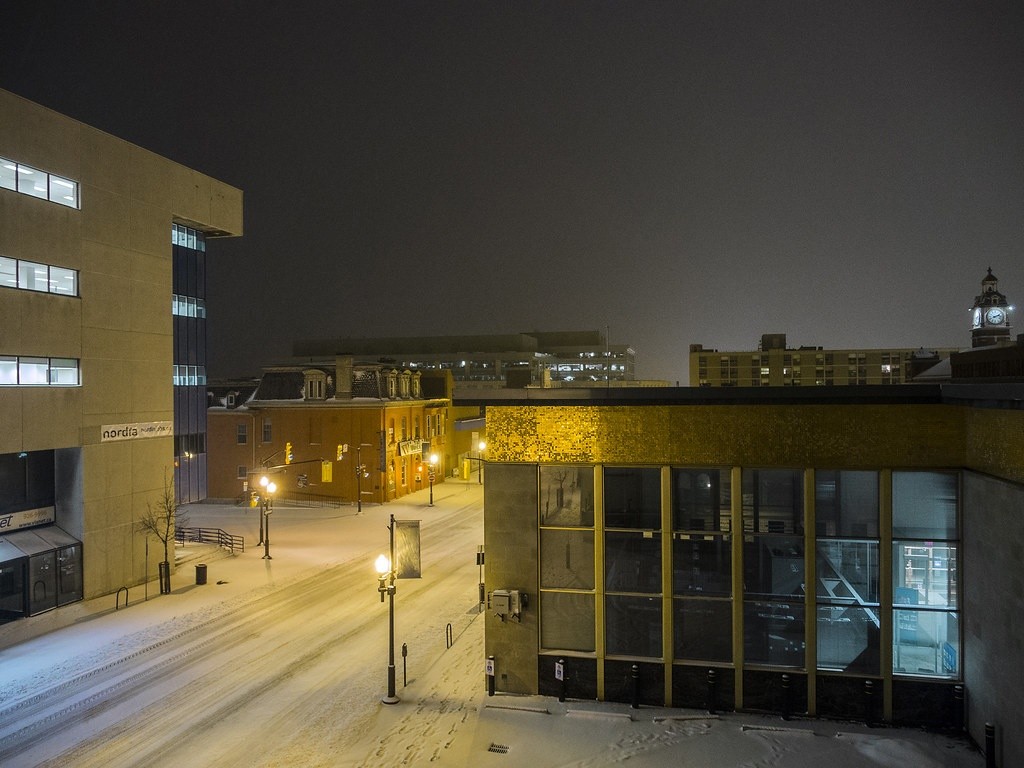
[427,454,437,507]
[262,482,275,560]
[257,476,267,547]
[377,514,402,704]
[478,442,486,484]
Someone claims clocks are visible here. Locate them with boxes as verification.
[986,308,1004,324]
[973,310,980,326]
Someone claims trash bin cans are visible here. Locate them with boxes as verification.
[195,564,207,585]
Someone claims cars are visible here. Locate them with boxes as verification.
[237,489,269,508]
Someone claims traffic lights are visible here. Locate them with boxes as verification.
[337,445,343,461]
[286,442,293,464]
